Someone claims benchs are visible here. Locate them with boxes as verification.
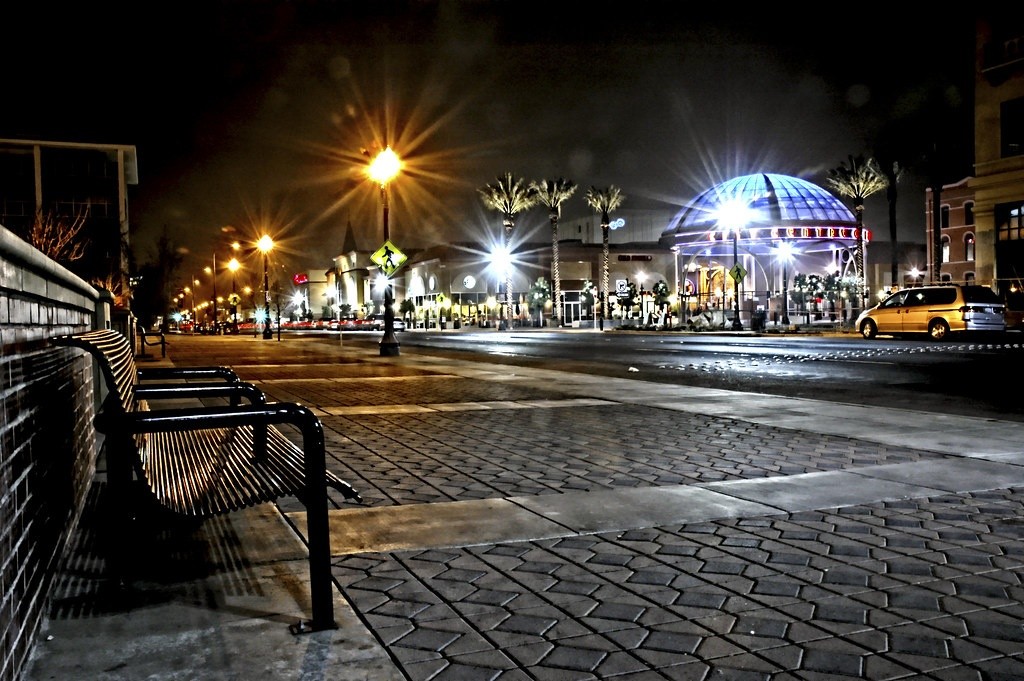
[139,326,170,359]
[46,329,365,636]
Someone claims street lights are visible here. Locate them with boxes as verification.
[258,235,279,339]
[366,147,405,356]
[187,266,213,331]
[226,257,240,333]
[773,243,798,323]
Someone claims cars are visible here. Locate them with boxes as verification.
[856,285,1009,340]
[179,316,405,336]
